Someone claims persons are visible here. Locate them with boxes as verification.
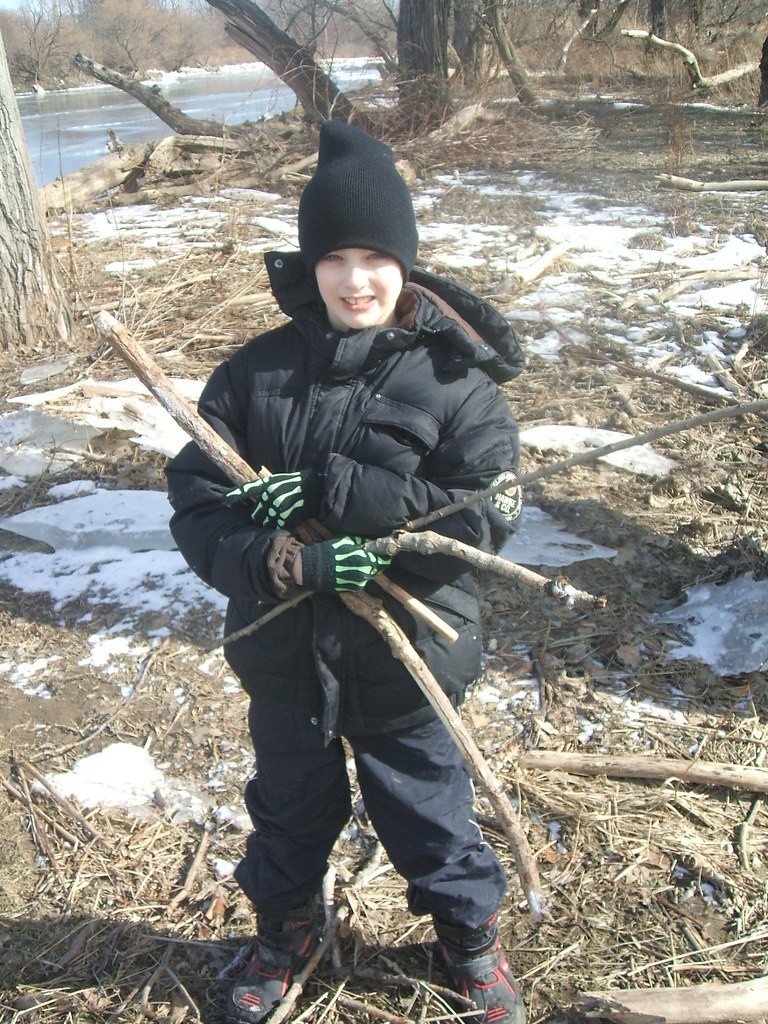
[169,123,525,1024]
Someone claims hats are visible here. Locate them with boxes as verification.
[298,121,418,288]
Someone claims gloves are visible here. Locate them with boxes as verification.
[224,468,322,530]
[299,535,393,593]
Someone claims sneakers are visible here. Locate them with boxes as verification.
[434,909,526,1024]
[223,889,325,1024]
